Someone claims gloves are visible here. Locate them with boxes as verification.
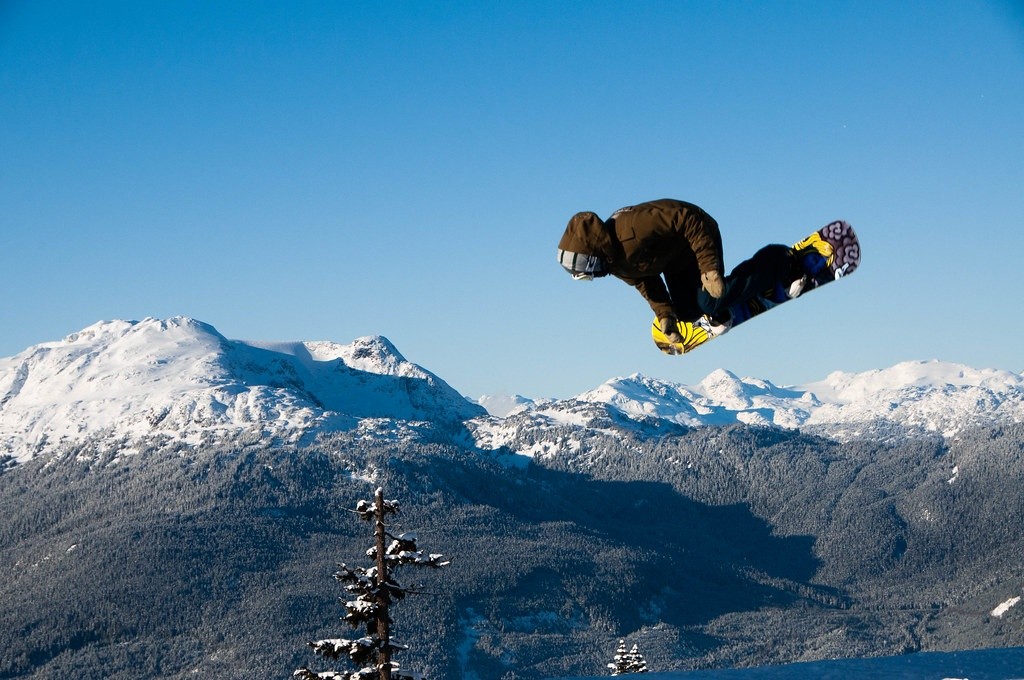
[659,318,685,343]
[702,271,725,299]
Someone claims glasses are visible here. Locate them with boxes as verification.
[572,272,594,281]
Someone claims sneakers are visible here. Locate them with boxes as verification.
[786,273,807,298]
[707,317,733,337]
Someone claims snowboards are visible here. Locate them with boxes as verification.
[650,219,864,360]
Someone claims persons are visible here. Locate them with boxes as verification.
[557,198,805,343]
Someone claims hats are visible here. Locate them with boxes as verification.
[557,248,601,273]
